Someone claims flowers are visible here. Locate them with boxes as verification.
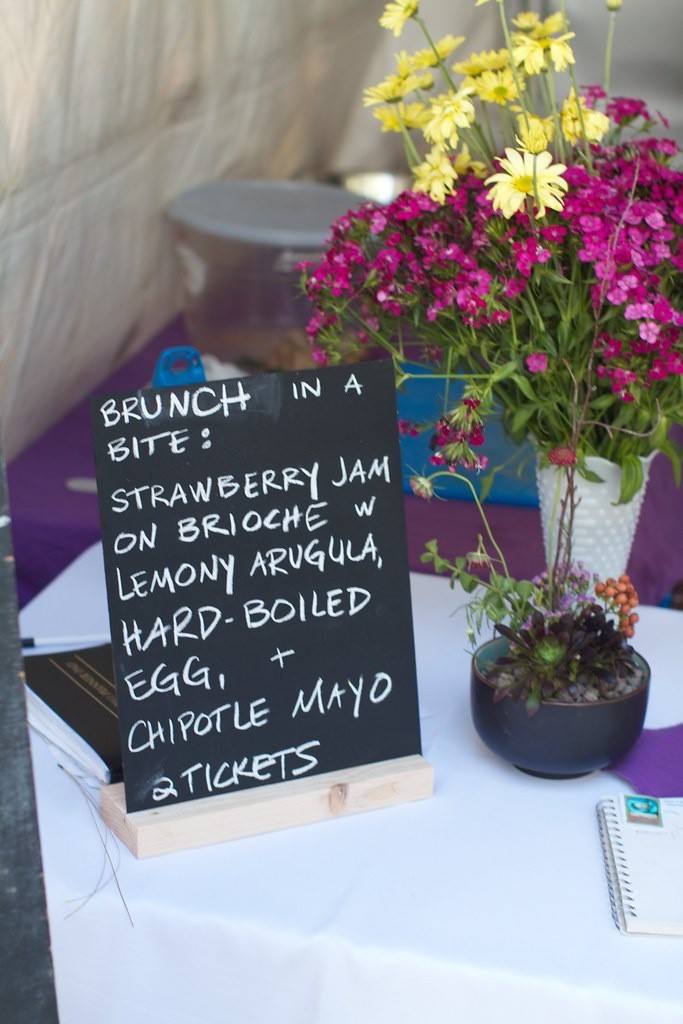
[288,1,683,504]
[406,454,640,717]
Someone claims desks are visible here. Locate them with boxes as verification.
[18,538,683,1024]
[1,312,683,611]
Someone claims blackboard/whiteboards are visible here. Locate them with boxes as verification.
[90,356,423,814]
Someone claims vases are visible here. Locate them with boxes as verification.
[532,440,658,614]
[474,635,649,780]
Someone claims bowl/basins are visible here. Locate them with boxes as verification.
[394,356,539,510]
[166,177,388,377]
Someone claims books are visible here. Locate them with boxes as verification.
[21,641,133,787]
[594,789,683,941]
